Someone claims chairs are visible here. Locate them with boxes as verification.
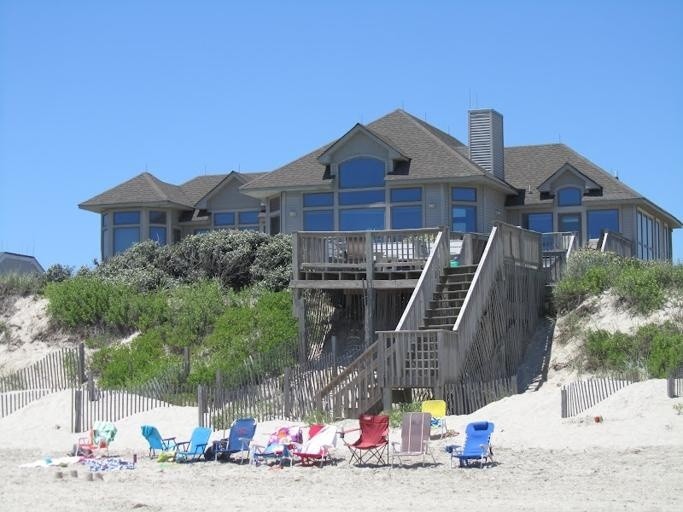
[445,421,495,470]
[172,427,212,464]
[338,414,389,468]
[140,425,177,458]
[248,426,303,469]
[76,421,116,458]
[214,417,257,464]
[291,423,337,467]
[391,412,439,468]
[421,402,451,441]
